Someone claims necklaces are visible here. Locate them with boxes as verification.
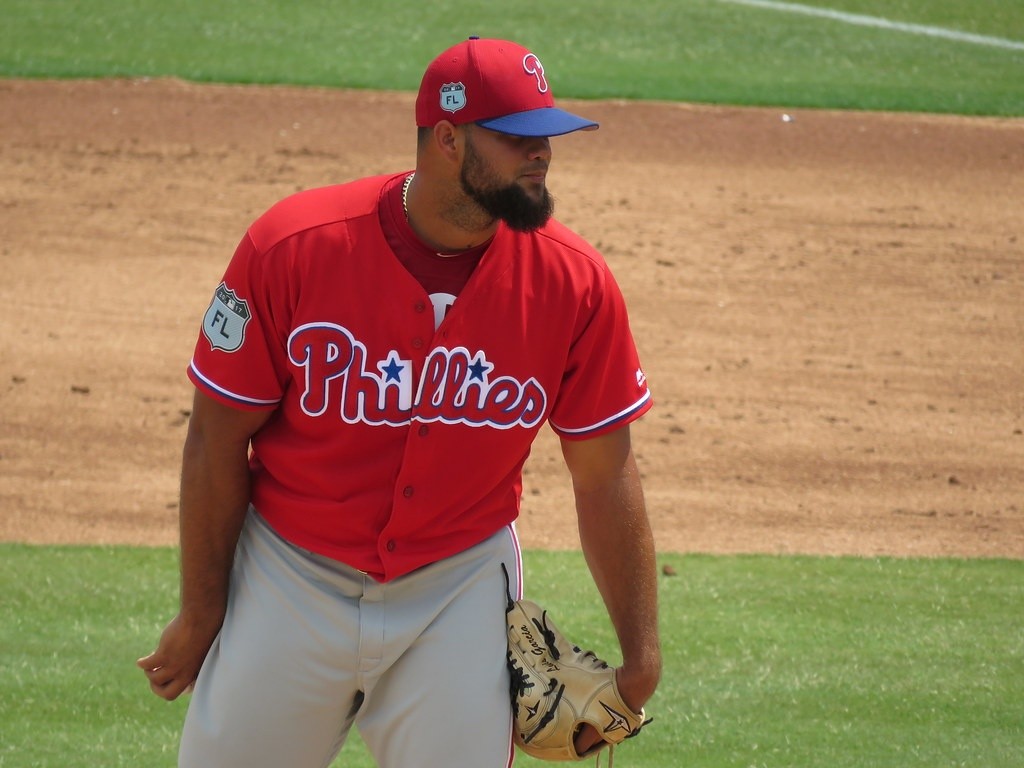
[402,173,415,222]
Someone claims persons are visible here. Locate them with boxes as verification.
[139,36,661,768]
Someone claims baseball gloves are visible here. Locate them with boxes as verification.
[501,557,655,768]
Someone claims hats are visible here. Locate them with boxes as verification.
[415,37,598,136]
